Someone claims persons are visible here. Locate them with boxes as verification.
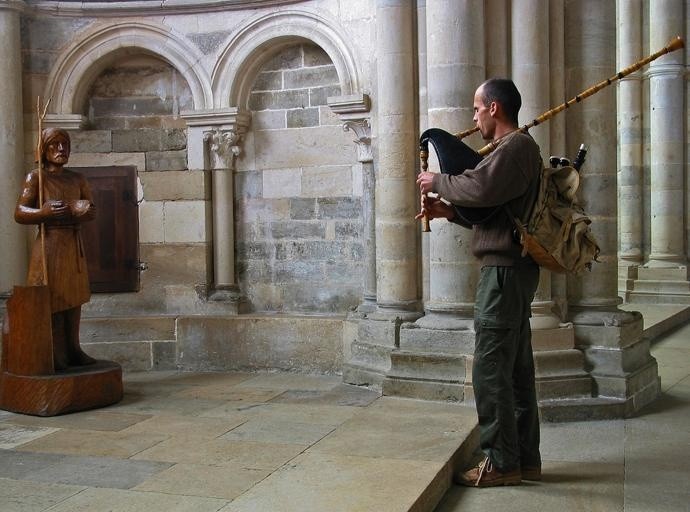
[13,124,100,372]
[413,76,544,490]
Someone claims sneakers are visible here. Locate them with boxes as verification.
[456,459,542,489]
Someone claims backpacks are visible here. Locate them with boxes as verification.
[512,166,602,276]
